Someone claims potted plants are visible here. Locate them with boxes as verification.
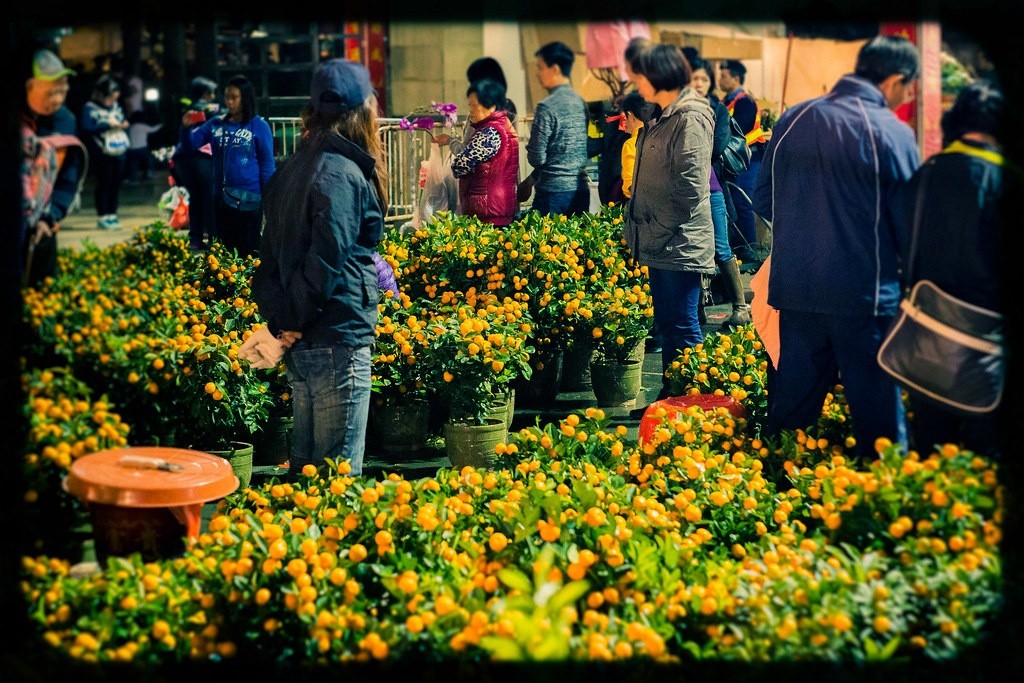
[19,201,1004,667]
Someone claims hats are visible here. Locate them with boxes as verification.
[191,77,218,94]
[24,49,76,81]
[310,58,370,113]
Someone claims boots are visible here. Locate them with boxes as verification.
[717,255,752,329]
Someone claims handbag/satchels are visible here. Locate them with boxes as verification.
[418,140,462,225]
[713,103,753,181]
[169,194,190,229]
[876,280,1003,413]
[102,130,130,155]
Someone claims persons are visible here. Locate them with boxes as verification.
[11,50,88,290]
[122,110,163,185]
[624,42,716,420]
[234,60,394,486]
[888,81,1010,465]
[173,78,274,258]
[78,75,132,231]
[429,38,767,329]
[752,35,921,471]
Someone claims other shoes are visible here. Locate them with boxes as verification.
[630,390,687,418]
[97,219,121,230]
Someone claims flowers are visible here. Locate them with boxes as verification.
[400,102,457,145]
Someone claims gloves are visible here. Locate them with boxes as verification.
[238,326,284,369]
